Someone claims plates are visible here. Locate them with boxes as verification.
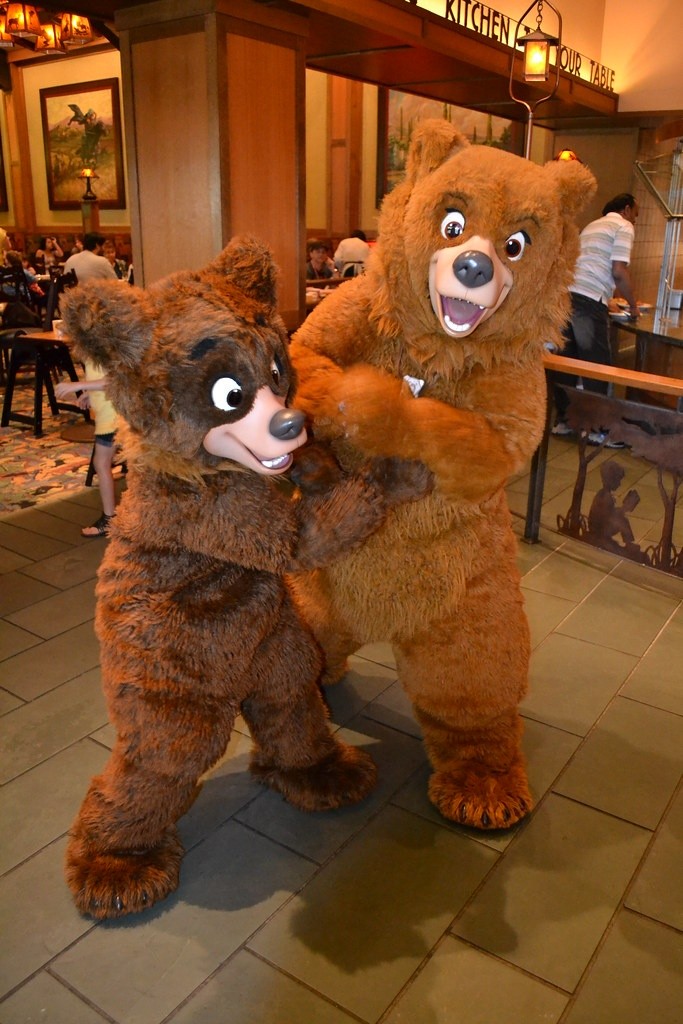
[618,303,654,311]
[609,310,643,321]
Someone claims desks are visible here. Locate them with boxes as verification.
[612,308,683,410]
[34,274,52,316]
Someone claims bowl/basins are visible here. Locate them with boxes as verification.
[306,287,334,298]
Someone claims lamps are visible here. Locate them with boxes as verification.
[507,0,563,160]
[78,166,100,199]
[0,0,95,56]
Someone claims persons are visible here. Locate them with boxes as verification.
[0,228,134,325]
[306,238,334,280]
[543,193,640,450]
[55,358,122,537]
[333,229,373,278]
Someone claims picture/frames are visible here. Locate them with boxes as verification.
[39,77,127,210]
[375,86,525,211]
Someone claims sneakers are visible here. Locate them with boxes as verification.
[551,417,573,435]
[581,429,625,448]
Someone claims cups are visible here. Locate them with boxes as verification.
[306,292,318,303]
[52,320,64,336]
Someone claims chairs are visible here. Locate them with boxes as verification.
[0,261,134,387]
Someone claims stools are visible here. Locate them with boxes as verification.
[0,331,91,438]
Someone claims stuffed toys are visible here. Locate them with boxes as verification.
[60,236,435,922]
[276,118,597,831]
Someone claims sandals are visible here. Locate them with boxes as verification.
[81,512,116,537]
